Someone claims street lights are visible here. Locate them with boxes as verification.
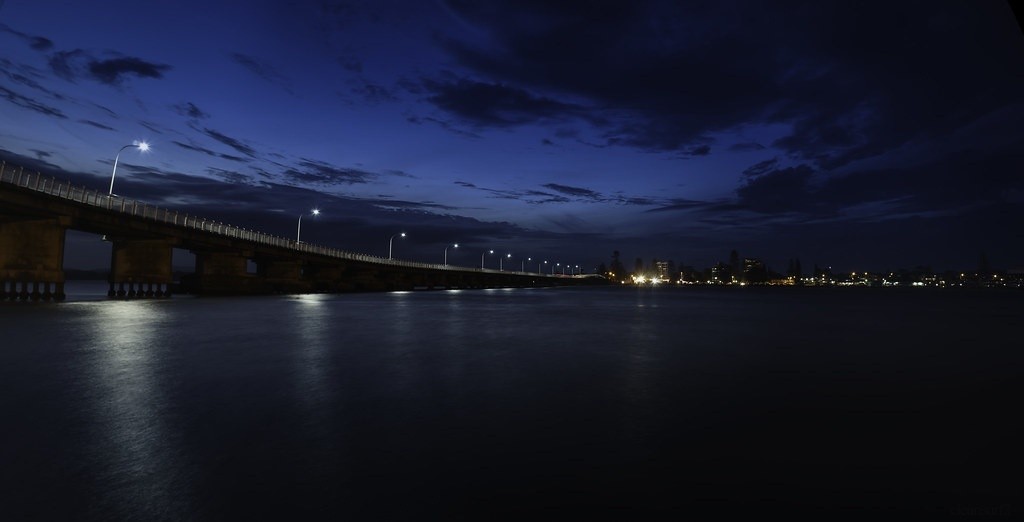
[538,260,547,273]
[444,242,459,264]
[297,209,320,245]
[500,253,512,270]
[389,233,406,260]
[522,256,532,270]
[552,262,559,274]
[562,264,569,274]
[481,249,494,268]
[108,143,148,199]
[571,264,578,274]
